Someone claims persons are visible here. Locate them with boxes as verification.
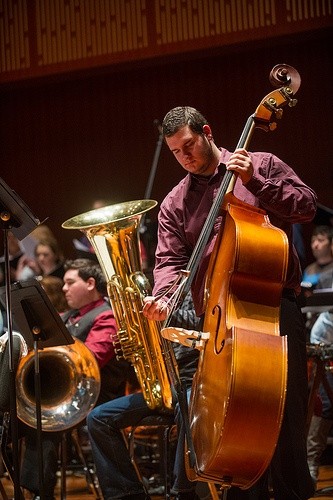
[143,106,317,500]
[293,225,333,287]
[87,291,201,499]
[0,199,146,317]
[306,308,333,480]
[20,258,122,500]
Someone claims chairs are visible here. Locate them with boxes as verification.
[58,414,175,500]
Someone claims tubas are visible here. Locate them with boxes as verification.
[61,198,181,410]
[15,335,102,433]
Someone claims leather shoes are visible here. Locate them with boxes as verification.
[33,496,40,500]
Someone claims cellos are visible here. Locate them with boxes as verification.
[184,63,301,491]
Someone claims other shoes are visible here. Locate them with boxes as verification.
[175,492,199,500]
